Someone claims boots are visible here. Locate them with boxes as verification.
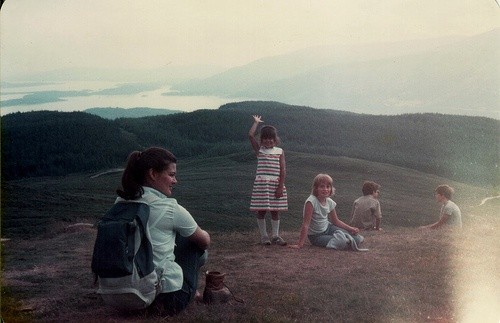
[202,271,233,305]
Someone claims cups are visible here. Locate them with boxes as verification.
[206,271,224,290]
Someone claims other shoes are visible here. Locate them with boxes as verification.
[271,235,287,245]
[260,233,271,245]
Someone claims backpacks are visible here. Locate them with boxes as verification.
[91,195,174,311]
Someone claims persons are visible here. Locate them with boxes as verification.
[248,115,288,246]
[95,147,211,314]
[417,185,462,246]
[285,174,368,252]
[348,181,383,231]
